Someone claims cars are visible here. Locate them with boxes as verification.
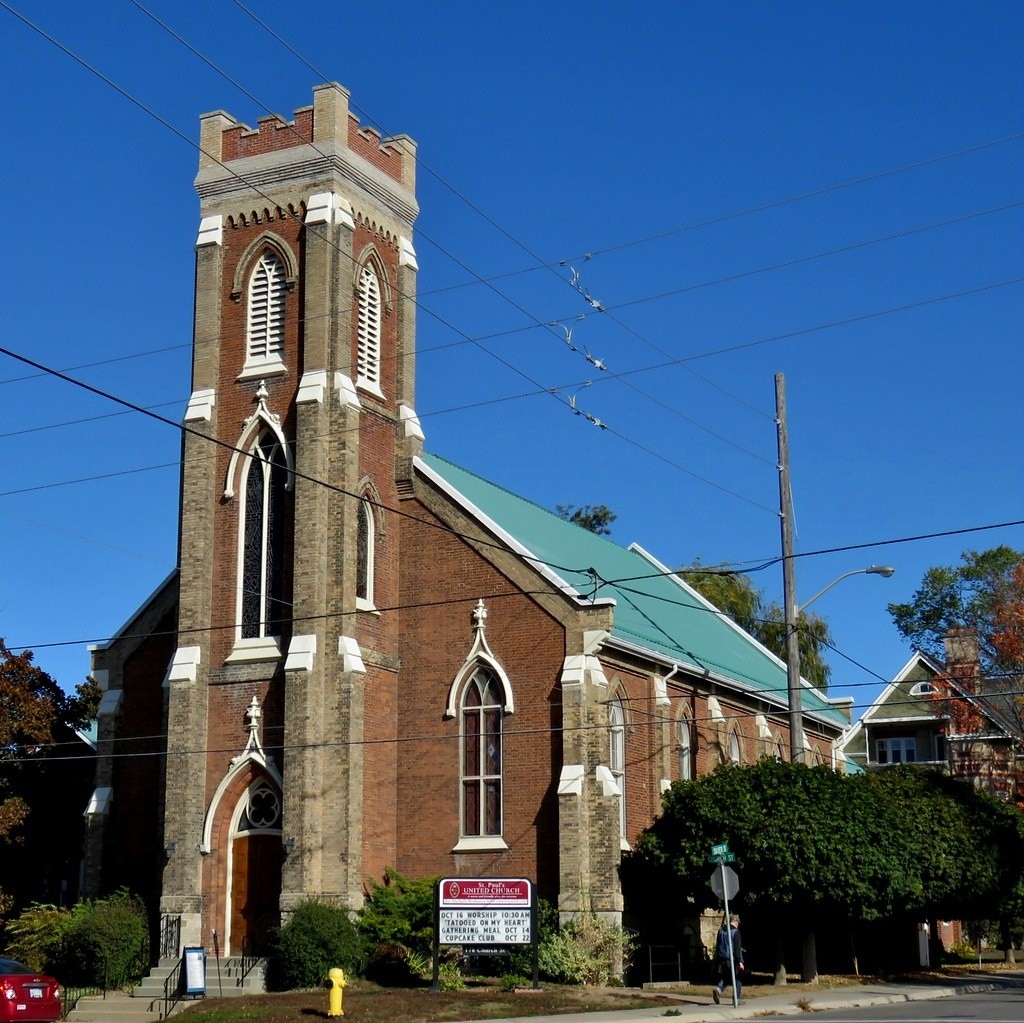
[0,958,61,1023]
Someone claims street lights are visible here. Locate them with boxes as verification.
[782,565,895,763]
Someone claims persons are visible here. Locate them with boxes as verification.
[712,915,745,1005]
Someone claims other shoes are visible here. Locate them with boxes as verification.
[738,1001,746,1005]
[713,988,720,1004]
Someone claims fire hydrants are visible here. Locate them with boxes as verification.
[324,968,348,1018]
